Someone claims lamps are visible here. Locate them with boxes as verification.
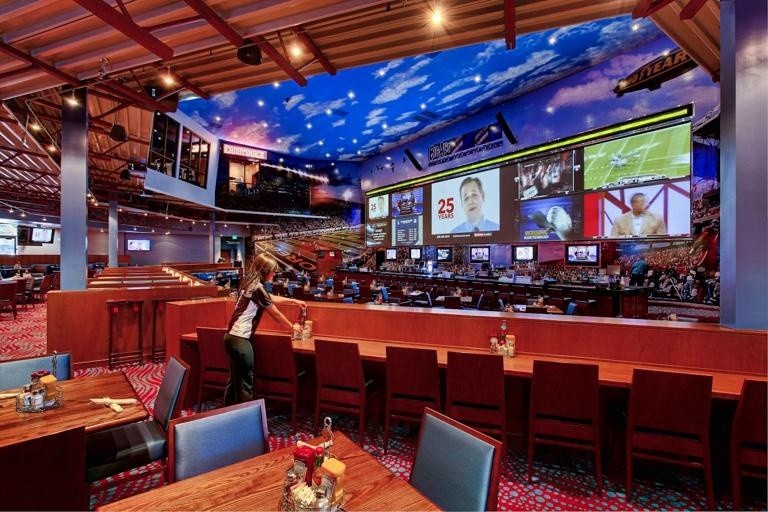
[109,123,127,142]
[236,35,260,66]
[119,170,130,180]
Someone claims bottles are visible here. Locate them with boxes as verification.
[300,308,308,326]
[500,319,506,346]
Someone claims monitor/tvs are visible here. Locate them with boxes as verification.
[566,243,600,267]
[437,248,453,263]
[29,228,55,244]
[386,249,398,261]
[409,249,422,258]
[513,246,537,262]
[469,245,491,264]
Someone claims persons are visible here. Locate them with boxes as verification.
[533,261,588,282]
[223,253,304,407]
[487,265,493,277]
[610,193,665,236]
[361,259,476,276]
[537,293,545,307]
[455,286,463,298]
[474,248,488,260]
[691,193,719,223]
[609,245,720,306]
[374,196,388,217]
[521,156,571,194]
[449,176,499,234]
[253,213,349,236]
[15,260,22,271]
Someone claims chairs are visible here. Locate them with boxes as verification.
[408,406,504,512]
[250,332,314,435]
[110,355,191,463]
[0,350,73,391]
[626,369,716,511]
[383,346,442,458]
[527,356,605,490]
[444,351,509,468]
[731,379,768,511]
[314,339,382,440]
[166,398,272,486]
[194,326,230,413]
[0,272,60,319]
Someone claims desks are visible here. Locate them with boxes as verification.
[0,371,150,511]
